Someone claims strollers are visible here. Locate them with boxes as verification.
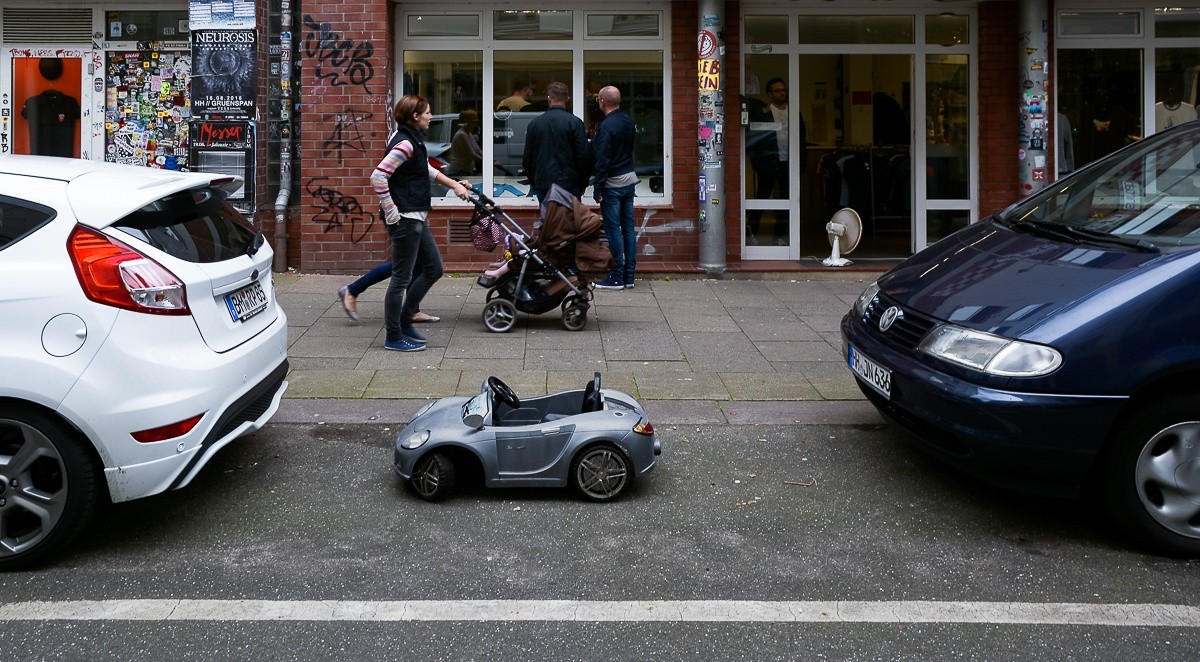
[458,180,610,331]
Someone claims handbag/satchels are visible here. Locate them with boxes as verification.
[469,199,500,252]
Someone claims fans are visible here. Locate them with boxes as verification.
[822,208,863,266]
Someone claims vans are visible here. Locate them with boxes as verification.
[407,111,545,177]
[837,116,1200,560]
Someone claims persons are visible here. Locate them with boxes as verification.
[494,74,639,291]
[446,110,503,175]
[1153,86,1200,174]
[1057,110,1075,176]
[338,95,474,351]
[747,76,807,246]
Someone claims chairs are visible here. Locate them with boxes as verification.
[541,371,601,423]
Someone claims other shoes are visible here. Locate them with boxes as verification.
[338,288,358,321]
[411,315,441,323]
[745,225,758,245]
[773,237,788,245]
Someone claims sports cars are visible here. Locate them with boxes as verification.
[392,371,661,503]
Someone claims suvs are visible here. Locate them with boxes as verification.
[1,152,288,569]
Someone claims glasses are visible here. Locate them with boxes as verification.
[527,86,536,93]
[771,88,786,93]
[596,98,605,102]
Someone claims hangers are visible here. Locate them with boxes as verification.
[1164,88,1180,105]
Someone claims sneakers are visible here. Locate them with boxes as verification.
[401,323,427,343]
[592,274,624,289]
[384,337,426,352]
[623,277,634,287]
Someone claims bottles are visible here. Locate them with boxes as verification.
[927,115,936,144]
